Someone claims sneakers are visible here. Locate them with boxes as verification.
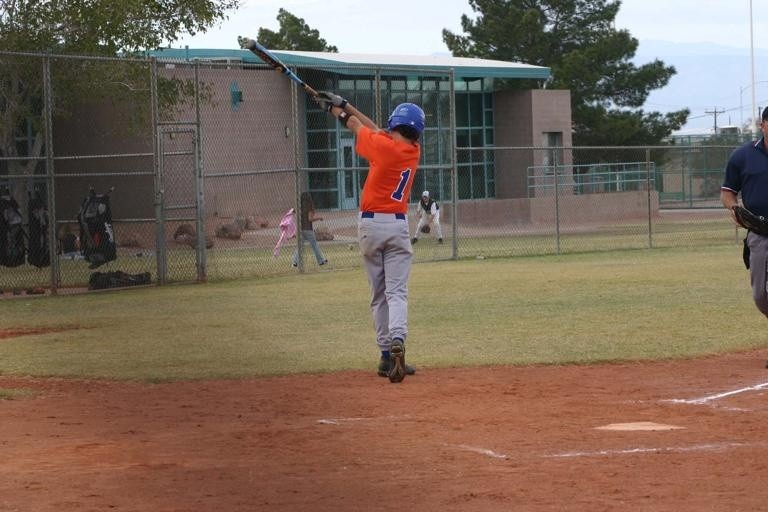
[377,339,416,383]
[411,238,417,244]
[319,260,327,265]
[438,239,442,243]
[292,263,297,268]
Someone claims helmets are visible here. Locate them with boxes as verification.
[387,103,425,134]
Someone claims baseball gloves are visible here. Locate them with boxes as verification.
[731,202,767,234]
[421,225,430,233]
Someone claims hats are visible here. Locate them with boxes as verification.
[422,191,429,197]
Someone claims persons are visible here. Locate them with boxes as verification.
[718,102,768,321]
[409,189,444,245]
[288,191,328,269]
[306,88,430,385]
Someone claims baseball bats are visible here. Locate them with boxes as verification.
[244,38,320,98]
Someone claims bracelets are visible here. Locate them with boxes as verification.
[337,110,352,127]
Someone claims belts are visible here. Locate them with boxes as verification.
[362,212,405,219]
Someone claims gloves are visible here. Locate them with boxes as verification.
[311,91,348,113]
